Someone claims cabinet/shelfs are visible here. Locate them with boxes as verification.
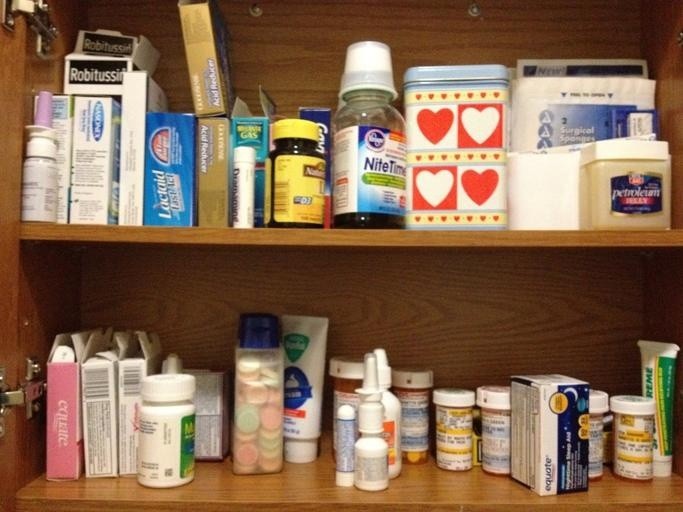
[0,0,683,511]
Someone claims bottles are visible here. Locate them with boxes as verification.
[389,361,433,466]
[354,352,390,494]
[137,312,284,488]
[476,384,511,476]
[610,395,656,480]
[586,388,610,479]
[429,388,476,473]
[19,89,59,222]
[373,348,403,481]
[331,355,364,469]
[335,403,357,488]
[229,41,408,228]
[472,407,483,467]
